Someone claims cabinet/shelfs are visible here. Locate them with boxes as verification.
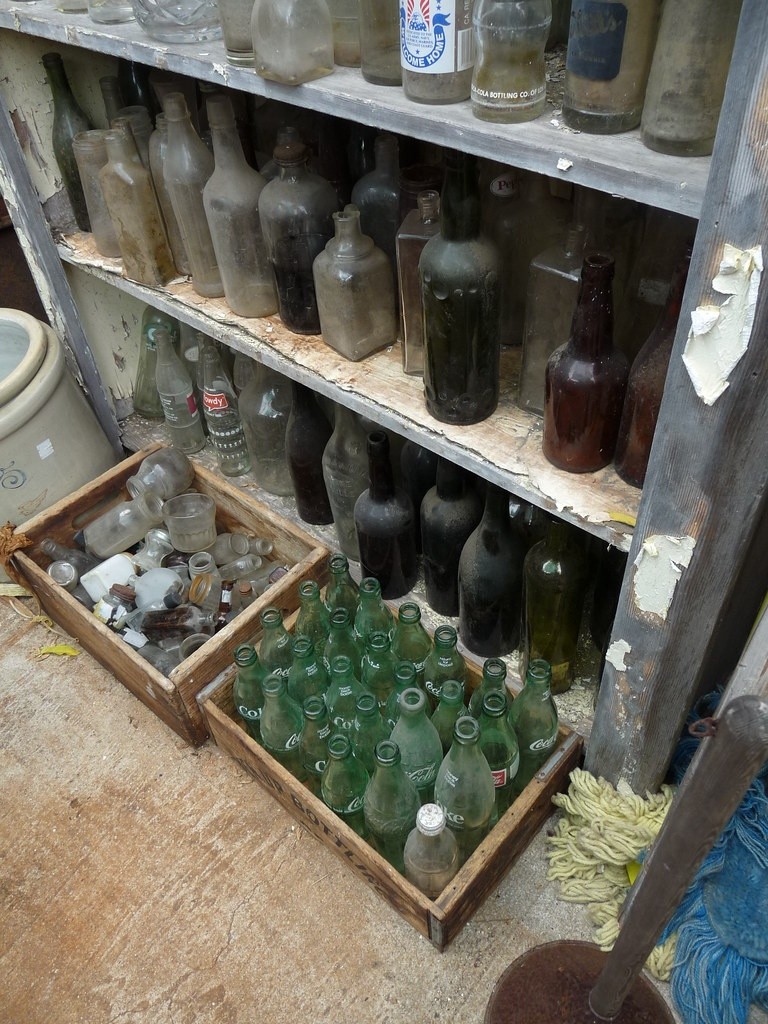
[0,1,768,786]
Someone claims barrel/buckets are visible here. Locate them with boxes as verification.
[0,307,116,527]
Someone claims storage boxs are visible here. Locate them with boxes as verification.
[195,578,583,953]
[4,442,328,748]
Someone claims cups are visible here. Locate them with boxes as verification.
[161,493,218,553]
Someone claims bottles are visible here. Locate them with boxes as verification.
[32,0,737,902]
